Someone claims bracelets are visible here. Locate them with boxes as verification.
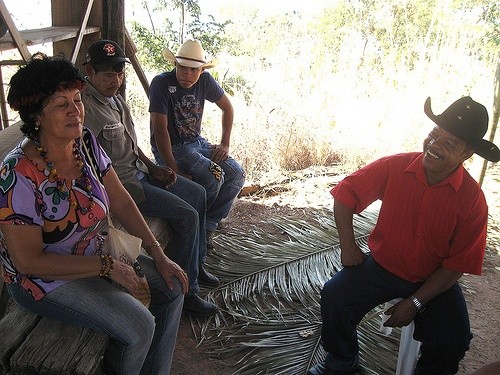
[144,240,160,255]
[99,254,114,278]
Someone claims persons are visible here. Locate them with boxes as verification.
[305,96,500,375]
[80,40,220,317]
[0,52,189,375]
[148,39,246,248]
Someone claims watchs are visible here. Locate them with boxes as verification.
[410,295,424,311]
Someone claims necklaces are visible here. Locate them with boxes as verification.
[34,136,95,212]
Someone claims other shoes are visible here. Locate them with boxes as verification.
[306,353,360,375]
[184,294,220,316]
[198,265,220,288]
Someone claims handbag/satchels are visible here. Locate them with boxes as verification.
[103,227,152,309]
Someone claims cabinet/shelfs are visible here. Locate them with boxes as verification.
[0,26,101,162]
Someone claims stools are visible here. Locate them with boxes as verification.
[380,298,420,375]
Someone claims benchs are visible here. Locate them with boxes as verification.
[0,208,172,375]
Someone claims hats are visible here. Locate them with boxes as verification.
[424,96,500,162]
[82,41,132,72]
[162,39,219,69]
